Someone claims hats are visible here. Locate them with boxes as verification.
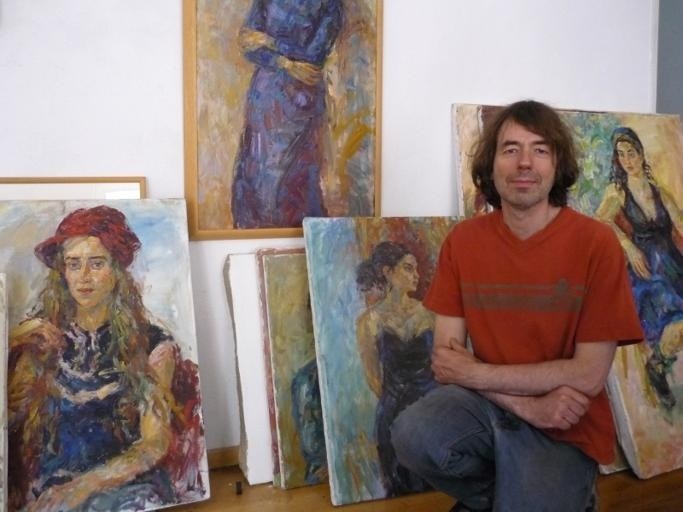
[33,206,142,276]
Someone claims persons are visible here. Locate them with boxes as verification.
[354,240,448,497]
[231,2,344,229]
[592,126,682,411]
[388,99,645,512]
[5,205,184,512]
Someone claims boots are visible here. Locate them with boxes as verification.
[644,344,677,409]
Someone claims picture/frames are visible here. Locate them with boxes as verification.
[0,177,146,199]
[182,0,383,241]
